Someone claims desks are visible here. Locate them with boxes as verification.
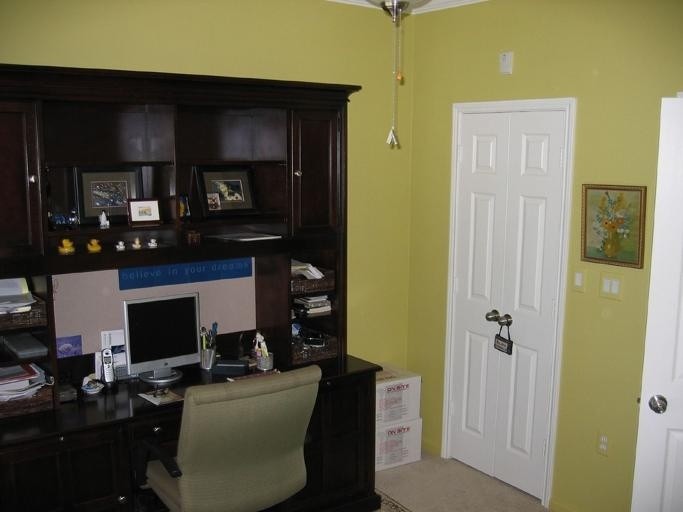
[0,325,376,512]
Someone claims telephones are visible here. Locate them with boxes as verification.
[102,386,118,421]
[101,349,119,389]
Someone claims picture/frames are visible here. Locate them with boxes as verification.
[579,183,648,270]
[194,162,260,219]
[71,164,144,225]
[124,197,162,229]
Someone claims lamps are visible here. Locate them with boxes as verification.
[367,1,412,23]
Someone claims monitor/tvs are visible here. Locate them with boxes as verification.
[120,291,202,383]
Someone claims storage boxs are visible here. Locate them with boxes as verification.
[372,419,426,473]
[376,365,423,426]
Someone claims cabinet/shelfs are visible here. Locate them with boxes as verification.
[31,62,295,241]
[294,76,361,239]
[0,61,38,262]
[0,276,59,434]
[280,241,348,370]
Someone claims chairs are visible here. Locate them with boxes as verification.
[137,364,323,508]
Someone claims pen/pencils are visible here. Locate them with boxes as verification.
[199,322,218,350]
